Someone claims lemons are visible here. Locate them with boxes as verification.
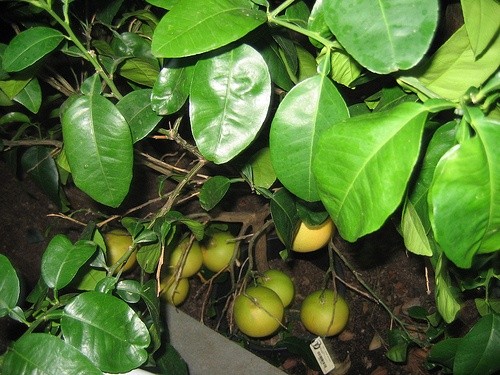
[102,215,349,338]
